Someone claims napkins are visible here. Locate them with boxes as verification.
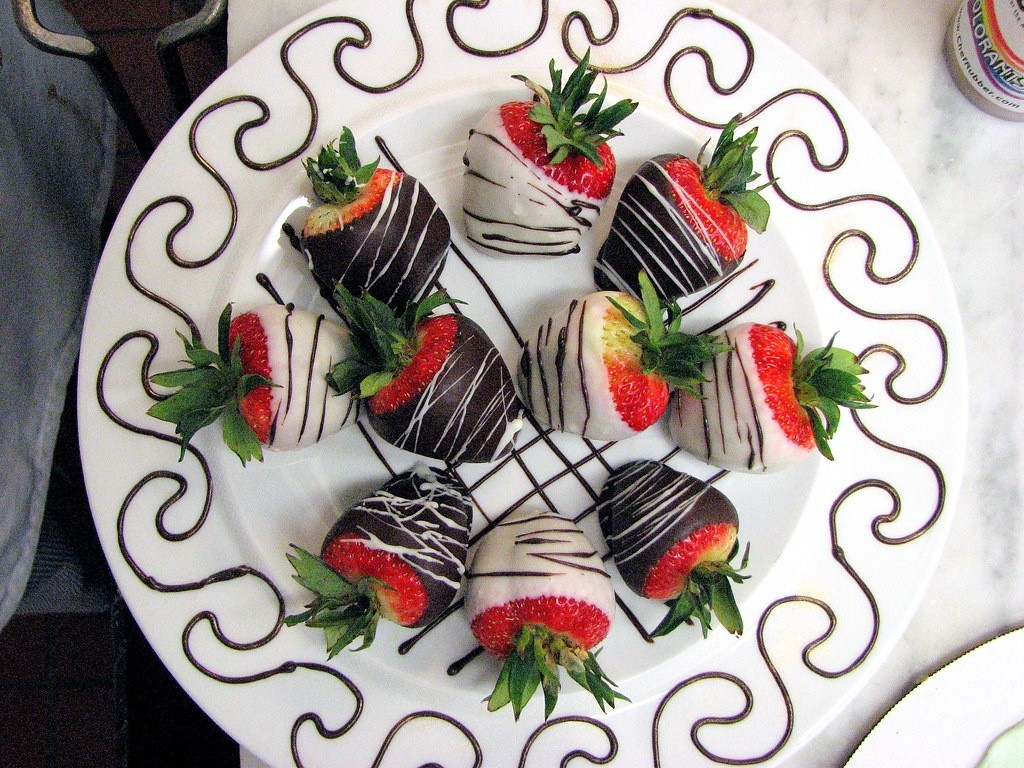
[0,1,118,634]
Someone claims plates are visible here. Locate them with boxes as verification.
[842,624,1024,768]
[76,2,960,767]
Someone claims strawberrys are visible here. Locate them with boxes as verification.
[145,45,878,722]
